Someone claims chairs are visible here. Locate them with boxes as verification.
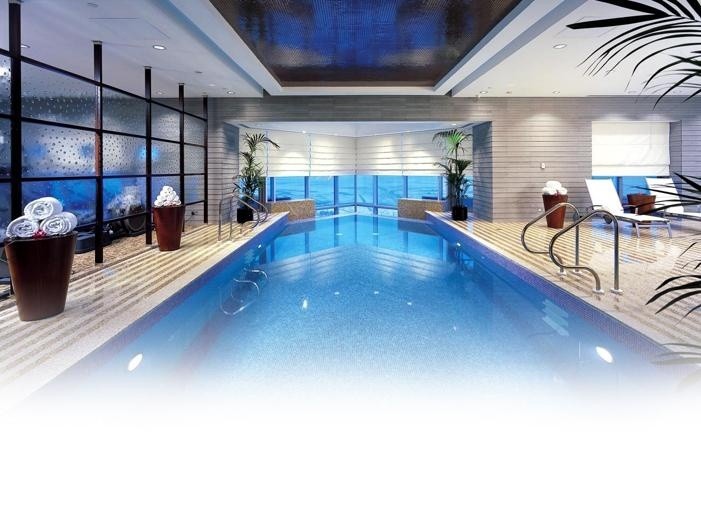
[573,178,701,238]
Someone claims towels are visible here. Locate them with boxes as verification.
[154,186,182,207]
[542,181,567,195]
[5,196,78,239]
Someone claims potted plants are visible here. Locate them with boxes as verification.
[231,132,282,224]
[431,128,484,220]
